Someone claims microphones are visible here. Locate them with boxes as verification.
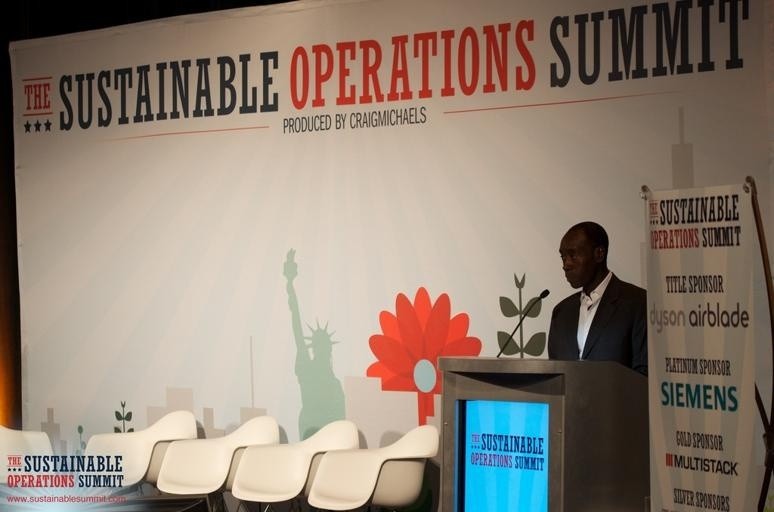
[491,286,551,357]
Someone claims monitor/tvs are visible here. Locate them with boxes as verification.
[458,400,549,512]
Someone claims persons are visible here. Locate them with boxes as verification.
[547,222,649,380]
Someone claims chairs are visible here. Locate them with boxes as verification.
[232,420,359,512]
[0,424,54,485]
[154,417,279,512]
[80,411,198,497]
[308,423,439,512]
